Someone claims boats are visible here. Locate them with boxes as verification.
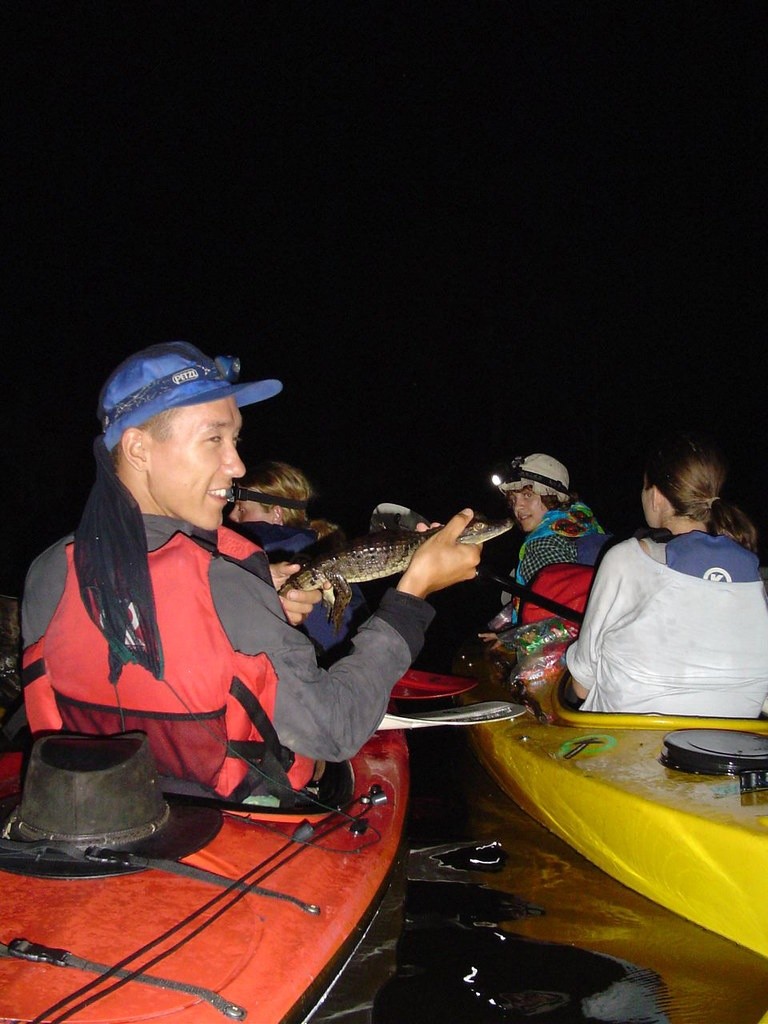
[437,665,768,962]
[0,700,412,1024]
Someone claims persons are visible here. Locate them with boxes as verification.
[568,437,768,717]
[488,453,622,637]
[220,462,374,670]
[24,340,483,811]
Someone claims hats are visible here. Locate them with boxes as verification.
[97,341,283,451]
[0,732,223,880]
[498,452,569,502]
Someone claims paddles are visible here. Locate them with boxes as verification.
[375,699,527,733]
[331,665,480,702]
[369,501,584,628]
